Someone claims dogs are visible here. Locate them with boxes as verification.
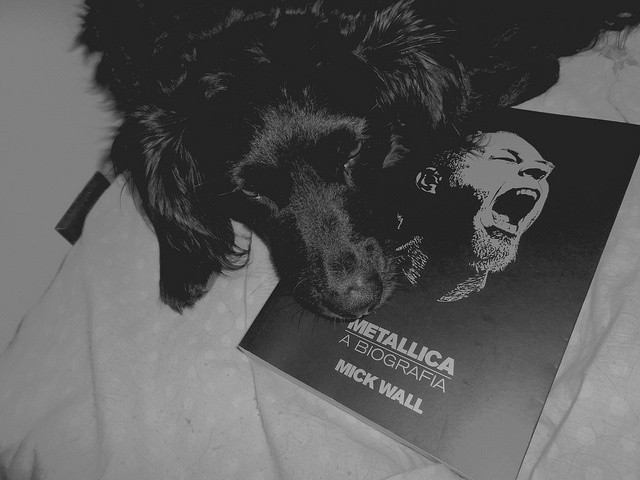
[75,1,640,321]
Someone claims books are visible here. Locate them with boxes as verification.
[236,109,640,480]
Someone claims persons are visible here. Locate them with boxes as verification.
[374,130,555,306]
